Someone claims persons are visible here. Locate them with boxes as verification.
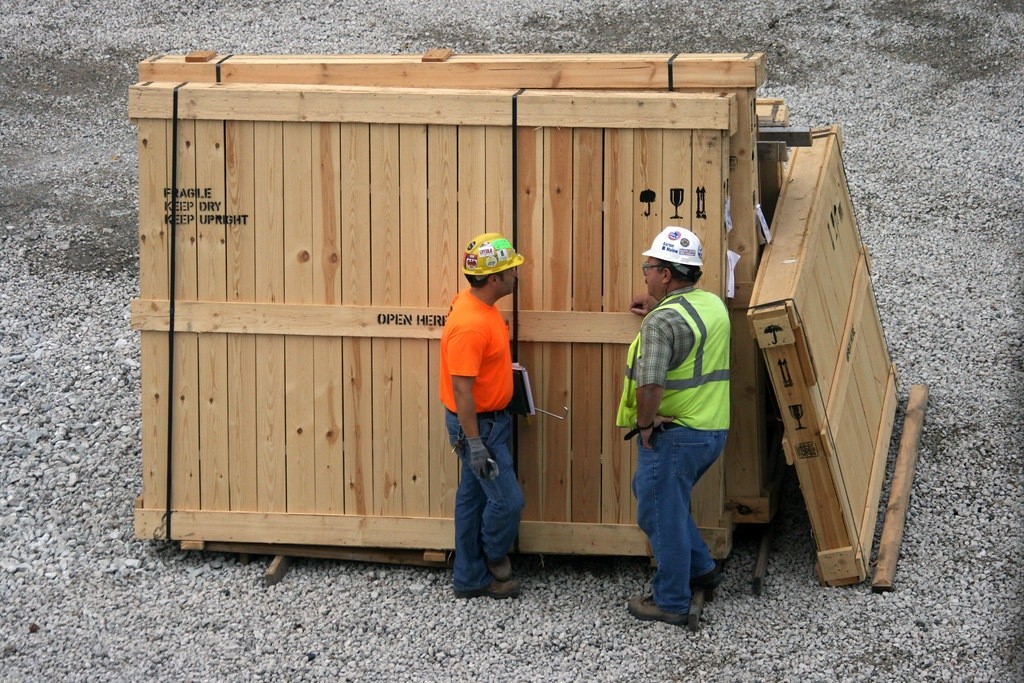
[438,230,529,602]
[615,225,732,627]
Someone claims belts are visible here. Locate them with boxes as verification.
[624,422,683,443]
[449,407,509,419]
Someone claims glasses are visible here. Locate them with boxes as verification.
[642,262,664,275]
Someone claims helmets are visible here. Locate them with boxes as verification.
[461,233,525,275]
[642,226,704,266]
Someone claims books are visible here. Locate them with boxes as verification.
[504,362,536,417]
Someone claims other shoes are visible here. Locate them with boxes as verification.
[629,594,688,625]
[454,579,520,600]
[690,567,721,589]
[485,556,512,581]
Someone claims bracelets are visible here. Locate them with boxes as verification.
[636,420,654,430]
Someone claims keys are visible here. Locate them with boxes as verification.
[451,440,459,453]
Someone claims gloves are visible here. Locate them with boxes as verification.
[467,437,491,477]
